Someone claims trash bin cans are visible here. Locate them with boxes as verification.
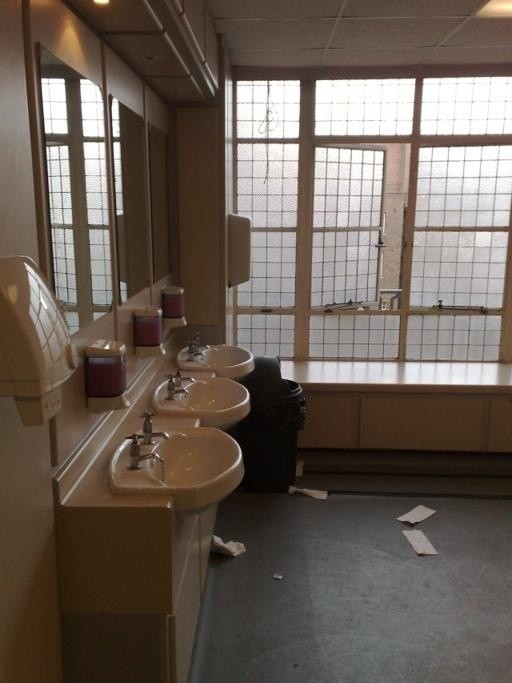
[228,356,306,494]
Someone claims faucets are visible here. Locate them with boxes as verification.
[193,334,209,352]
[187,341,206,361]
[140,410,169,445]
[126,434,165,470]
[165,374,190,400]
[174,367,196,387]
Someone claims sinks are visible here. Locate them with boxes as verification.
[176,345,254,380]
[153,377,250,432]
[109,427,244,515]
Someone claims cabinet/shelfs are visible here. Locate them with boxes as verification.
[54,502,223,683]
[280,360,512,452]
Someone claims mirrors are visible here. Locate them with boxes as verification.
[148,123,179,282]
[36,42,116,336]
[107,91,153,306]
